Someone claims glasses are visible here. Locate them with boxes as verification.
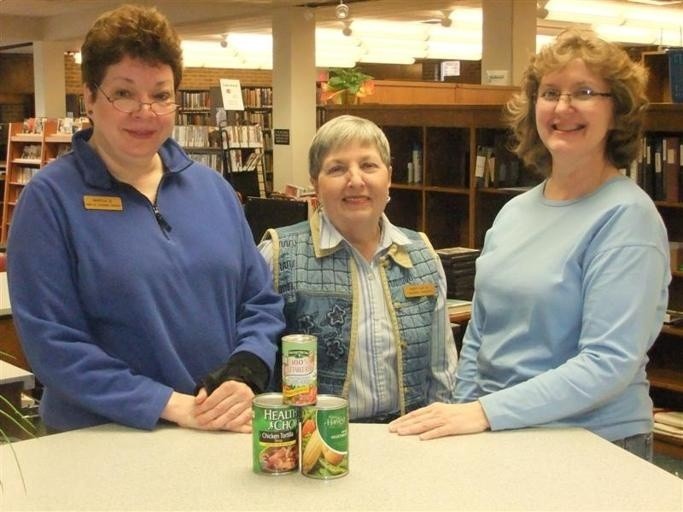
[532,87,616,100]
[88,78,181,116]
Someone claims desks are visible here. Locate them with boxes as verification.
[0,410,683,512]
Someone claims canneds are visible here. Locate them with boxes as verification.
[301,394,349,479]
[251,392,299,477]
[281,334,318,407]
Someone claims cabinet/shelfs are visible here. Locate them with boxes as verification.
[22,83,274,194]
[316,103,682,444]
[0,115,266,276]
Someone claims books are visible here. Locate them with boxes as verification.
[2,86,271,213]
[618,136,683,203]
[652,406,683,439]
[406,141,423,186]
[436,247,479,302]
[472,135,518,189]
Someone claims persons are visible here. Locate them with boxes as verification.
[257,109,463,428]
[384,28,676,466]
[6,3,288,438]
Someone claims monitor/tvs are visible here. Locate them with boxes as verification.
[245,194,310,247]
